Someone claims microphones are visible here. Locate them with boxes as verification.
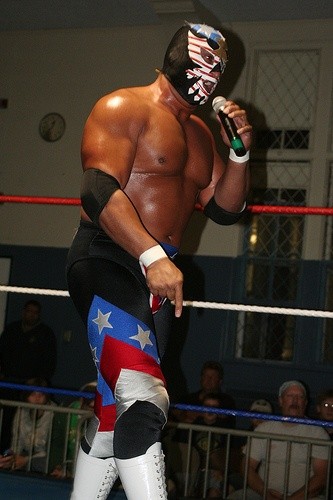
[212,96,246,157]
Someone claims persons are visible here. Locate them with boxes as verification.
[66,19,253,500]
[0,378,98,481]
[0,300,57,454]
[161,360,333,500]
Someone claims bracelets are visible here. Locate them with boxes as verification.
[139,244,167,268]
[228,147,249,164]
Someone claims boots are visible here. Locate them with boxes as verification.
[70,440,118,499]
[112,440,168,500]
